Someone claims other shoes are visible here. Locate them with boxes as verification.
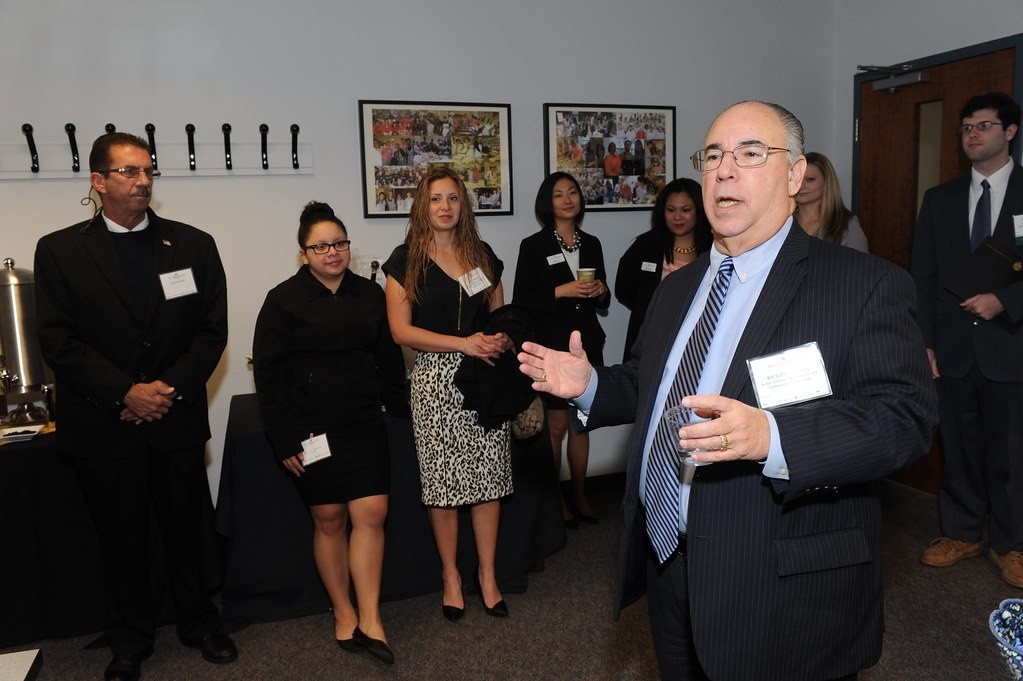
[352,625,394,663]
[335,636,364,653]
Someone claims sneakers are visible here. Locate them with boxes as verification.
[988,546,1023,587]
[921,537,984,566]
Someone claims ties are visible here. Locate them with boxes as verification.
[645,258,734,565]
[971,180,992,251]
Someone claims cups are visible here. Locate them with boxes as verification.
[665,405,714,466]
[577,268,595,294]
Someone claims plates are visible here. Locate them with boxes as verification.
[0,425,45,442]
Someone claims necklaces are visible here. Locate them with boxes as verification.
[672,243,697,254]
[554,229,581,253]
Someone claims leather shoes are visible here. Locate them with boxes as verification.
[177,627,238,664]
[103,656,141,681]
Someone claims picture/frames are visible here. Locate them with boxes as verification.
[359,99,514,220]
[542,102,677,212]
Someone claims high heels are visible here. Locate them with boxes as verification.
[477,570,509,616]
[443,569,465,623]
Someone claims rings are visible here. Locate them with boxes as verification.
[720,434,729,454]
[542,374,547,383]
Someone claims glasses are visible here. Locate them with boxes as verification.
[304,239,350,254]
[690,142,791,172]
[99,166,161,180]
[958,120,1003,134]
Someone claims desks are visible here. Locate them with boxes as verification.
[0,421,200,648]
[215,389,570,626]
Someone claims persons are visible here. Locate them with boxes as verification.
[794,152,867,251]
[511,171,612,530]
[614,179,716,364]
[371,109,501,210]
[380,166,511,620]
[556,111,666,205]
[251,201,408,666]
[918,94,1023,589]
[33,131,239,680]
[530,99,937,681]
